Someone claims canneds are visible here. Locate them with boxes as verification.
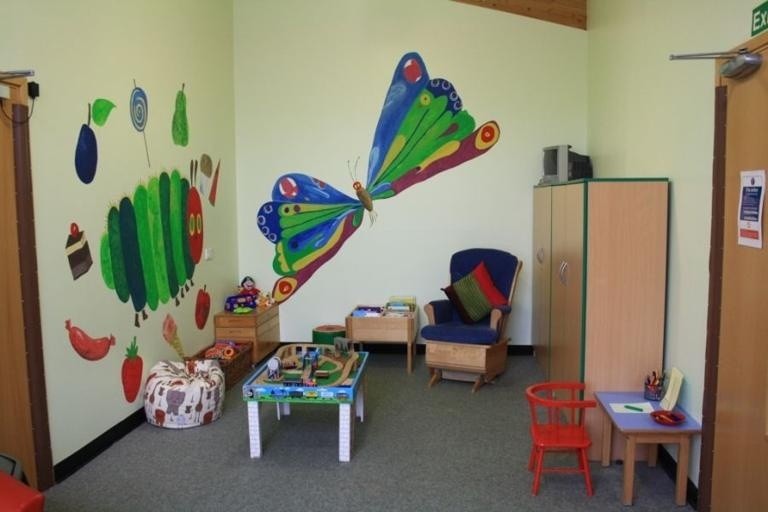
[302,351,319,383]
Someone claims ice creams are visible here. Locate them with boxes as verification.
[160,313,186,360]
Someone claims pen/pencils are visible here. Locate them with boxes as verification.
[656,414,681,423]
[624,405,643,412]
[645,368,666,401]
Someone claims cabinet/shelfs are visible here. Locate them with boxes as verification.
[531,177,670,462]
[346,305,417,374]
[214,301,280,371]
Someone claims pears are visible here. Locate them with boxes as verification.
[168,83,193,148]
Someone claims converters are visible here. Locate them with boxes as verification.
[28,81,39,98]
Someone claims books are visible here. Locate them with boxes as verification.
[352,295,416,318]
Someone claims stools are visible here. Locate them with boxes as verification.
[313,324,347,345]
[144,358,226,428]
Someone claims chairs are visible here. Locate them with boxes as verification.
[421,248,523,393]
[526,382,598,497]
[0,453,22,480]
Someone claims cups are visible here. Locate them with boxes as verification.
[645,384,663,400]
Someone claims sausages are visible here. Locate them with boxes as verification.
[65,319,118,362]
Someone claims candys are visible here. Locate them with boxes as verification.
[129,87,157,167]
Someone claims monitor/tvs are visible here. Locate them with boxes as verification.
[541,144,593,186]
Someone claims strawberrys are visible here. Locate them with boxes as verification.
[120,336,143,402]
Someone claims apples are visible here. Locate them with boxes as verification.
[194,284,211,330]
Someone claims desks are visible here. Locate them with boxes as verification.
[591,389,702,506]
[243,352,370,463]
[0,470,46,511]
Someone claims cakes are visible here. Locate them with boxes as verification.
[62,222,94,281]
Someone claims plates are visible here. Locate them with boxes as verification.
[650,410,686,425]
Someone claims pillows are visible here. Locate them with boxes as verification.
[440,262,507,325]
